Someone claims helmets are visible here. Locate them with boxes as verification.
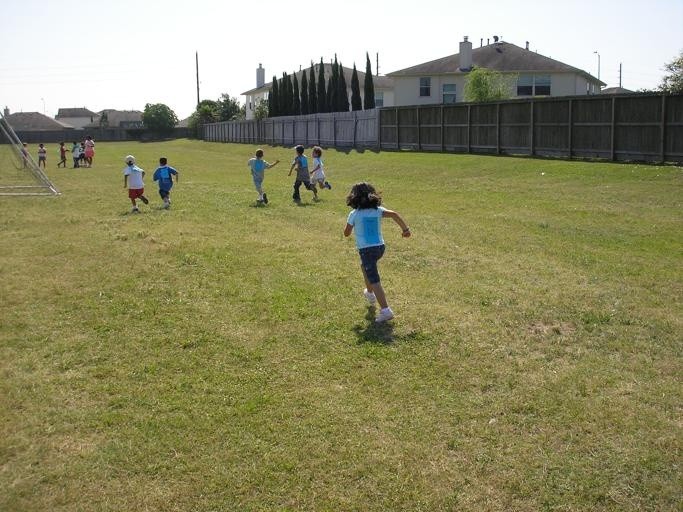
[124,154,134,164]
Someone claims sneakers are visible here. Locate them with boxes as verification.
[132,195,148,212]
[374,307,394,322]
[324,181,331,190]
[362,287,375,303]
[256,192,267,203]
[163,198,170,209]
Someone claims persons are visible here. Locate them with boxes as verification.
[152,156,179,210]
[56,141,69,169]
[344,182,412,322]
[83,135,95,168]
[78,142,85,167]
[123,154,148,213]
[20,142,28,170]
[37,144,46,169]
[307,146,332,201]
[247,149,280,205]
[70,141,80,169]
[287,145,317,203]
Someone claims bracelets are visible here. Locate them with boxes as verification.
[402,226,410,232]
